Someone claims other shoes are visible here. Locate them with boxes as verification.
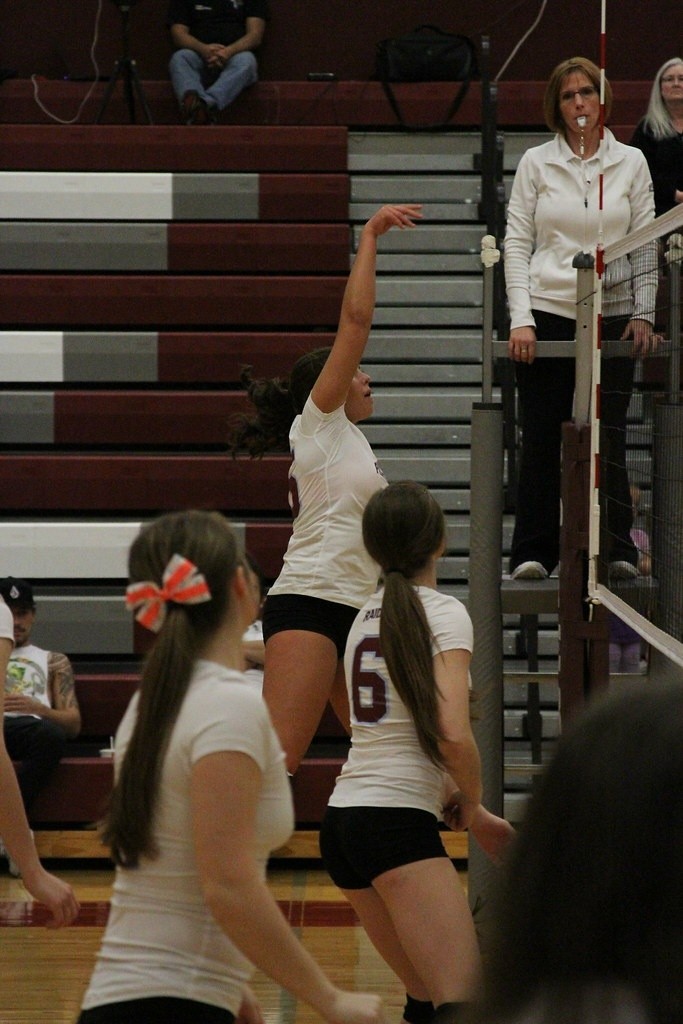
[609,559,640,580]
[510,561,547,580]
[11,828,35,879]
[183,91,212,126]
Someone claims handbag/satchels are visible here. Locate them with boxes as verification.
[376,23,479,134]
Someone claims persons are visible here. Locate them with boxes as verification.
[319,480,516,1022]
[460,678,683,1024]
[501,58,656,579]
[633,58,683,419]
[608,483,651,673]
[77,514,384,1024]
[0,583,80,929]
[230,203,425,774]
[168,0,267,125]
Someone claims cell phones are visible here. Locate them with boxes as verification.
[308,72,335,81]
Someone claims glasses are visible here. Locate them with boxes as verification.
[557,86,597,103]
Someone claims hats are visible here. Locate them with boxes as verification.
[0,576,35,610]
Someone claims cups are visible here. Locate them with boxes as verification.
[99,749,114,759]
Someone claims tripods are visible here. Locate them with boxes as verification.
[93,7,155,125]
